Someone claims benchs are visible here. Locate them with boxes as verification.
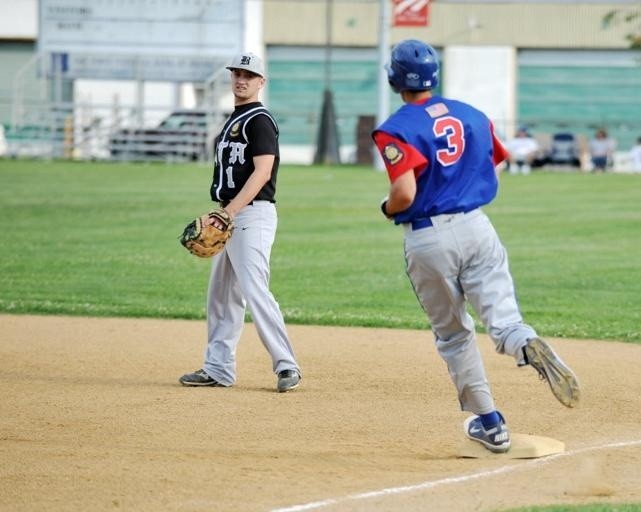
[268,59,640,154]
[1,47,233,165]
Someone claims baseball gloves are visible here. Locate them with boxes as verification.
[177,208,235,258]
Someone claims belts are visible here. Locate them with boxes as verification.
[218,199,276,210]
[409,205,478,233]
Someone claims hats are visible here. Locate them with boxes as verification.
[225,49,266,80]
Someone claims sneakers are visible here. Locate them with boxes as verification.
[276,367,301,393]
[461,413,512,454]
[180,368,218,387]
[525,337,583,409]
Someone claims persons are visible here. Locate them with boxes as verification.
[368,40,579,456]
[503,125,540,174]
[624,137,641,176]
[175,49,304,394]
[590,128,617,173]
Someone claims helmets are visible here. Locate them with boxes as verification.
[383,38,440,93]
